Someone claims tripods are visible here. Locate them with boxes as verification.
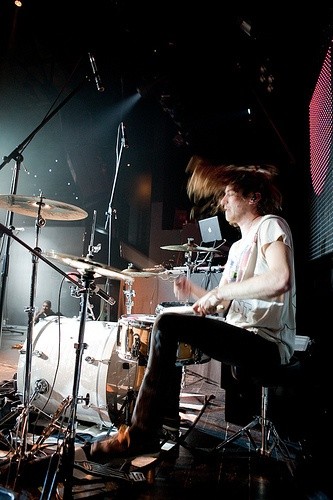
[0,205,90,461]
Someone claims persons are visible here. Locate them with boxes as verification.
[85,168,295,465]
[34,301,54,325]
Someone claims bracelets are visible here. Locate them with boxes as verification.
[215,286,220,301]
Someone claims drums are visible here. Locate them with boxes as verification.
[17,316,139,429]
[117,313,211,365]
[154,301,203,365]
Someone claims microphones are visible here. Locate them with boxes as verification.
[76,274,115,305]
[89,53,104,92]
[121,122,128,148]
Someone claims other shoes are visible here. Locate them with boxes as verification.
[89,426,161,462]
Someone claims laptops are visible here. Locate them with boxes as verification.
[198,216,223,243]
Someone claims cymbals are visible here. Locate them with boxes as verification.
[160,240,222,259]
[44,248,136,284]
[0,194,88,222]
